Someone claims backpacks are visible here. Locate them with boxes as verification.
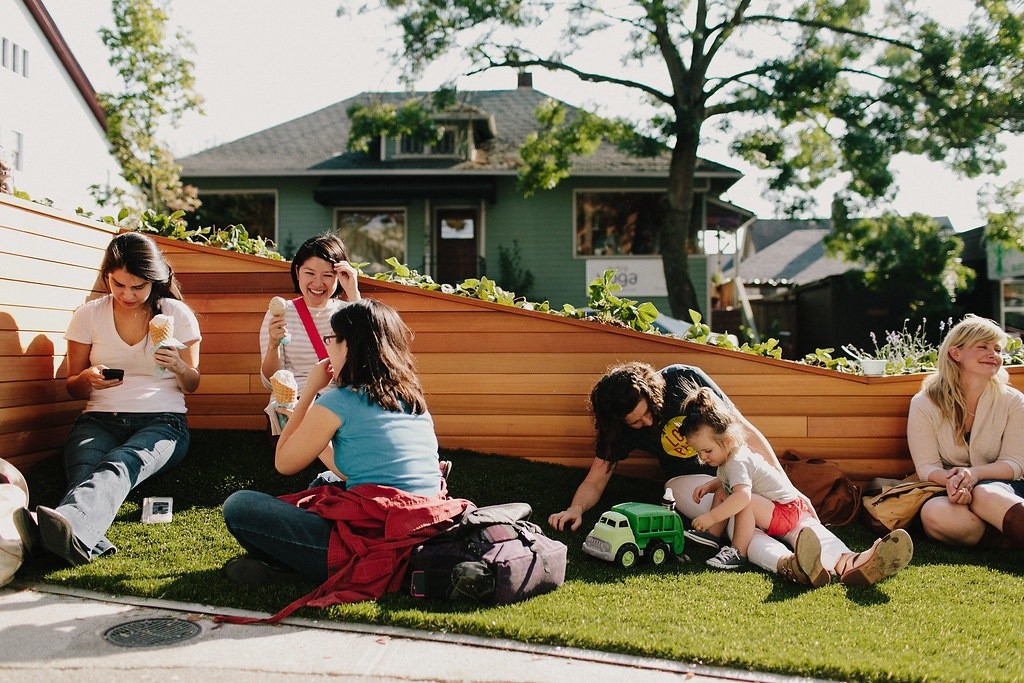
[401,504,568,607]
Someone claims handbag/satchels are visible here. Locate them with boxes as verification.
[777,449,862,526]
[861,473,947,535]
[0,458,29,588]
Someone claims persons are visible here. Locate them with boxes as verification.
[906,314,1024,549]
[548,361,914,589]
[679,387,803,569]
[36,231,203,566]
[222,299,442,586]
[260,234,452,497]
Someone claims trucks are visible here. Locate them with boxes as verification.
[581,502,685,569]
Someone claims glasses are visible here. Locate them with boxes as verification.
[324,335,336,345]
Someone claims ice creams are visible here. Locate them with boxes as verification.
[270,370,299,403]
[149,314,175,346]
[269,296,289,329]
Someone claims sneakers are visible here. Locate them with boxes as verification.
[683,528,720,550]
[706,545,749,570]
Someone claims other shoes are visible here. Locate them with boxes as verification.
[13,508,54,574]
[36,506,90,568]
[226,558,305,589]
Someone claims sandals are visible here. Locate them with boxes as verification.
[782,527,831,589]
[840,529,913,591]
[440,460,452,479]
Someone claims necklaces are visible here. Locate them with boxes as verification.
[968,412,975,417]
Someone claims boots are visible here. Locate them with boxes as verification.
[1003,501,1024,549]
[974,521,1016,551]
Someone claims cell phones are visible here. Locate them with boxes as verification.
[101,368,124,383]
